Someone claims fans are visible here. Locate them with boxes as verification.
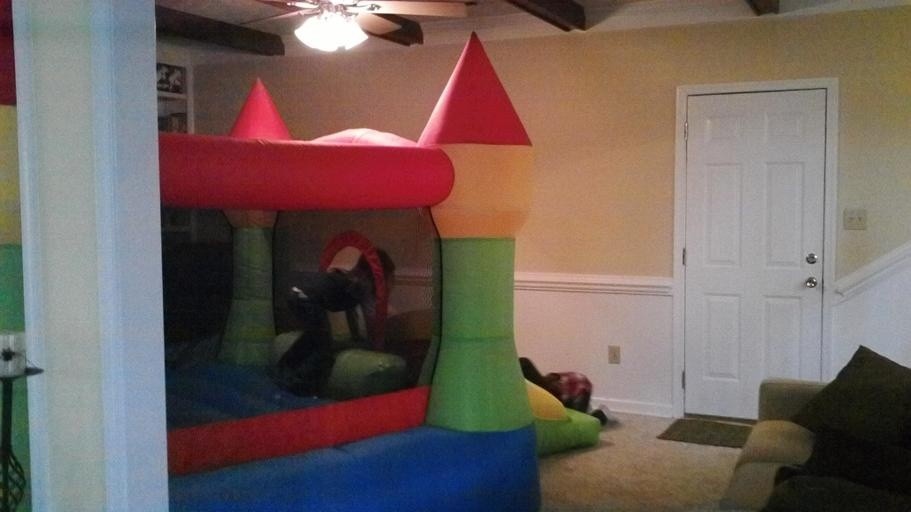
[238,0,466,47]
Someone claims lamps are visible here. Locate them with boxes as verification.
[288,8,370,56]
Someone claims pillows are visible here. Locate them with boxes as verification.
[761,461,909,511]
[792,342,909,437]
[795,415,910,491]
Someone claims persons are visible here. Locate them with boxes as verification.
[518,357,619,427]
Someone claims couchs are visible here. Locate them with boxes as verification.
[715,374,909,511]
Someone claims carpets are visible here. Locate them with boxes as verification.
[654,413,753,457]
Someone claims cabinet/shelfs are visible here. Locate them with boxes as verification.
[155,40,197,132]
